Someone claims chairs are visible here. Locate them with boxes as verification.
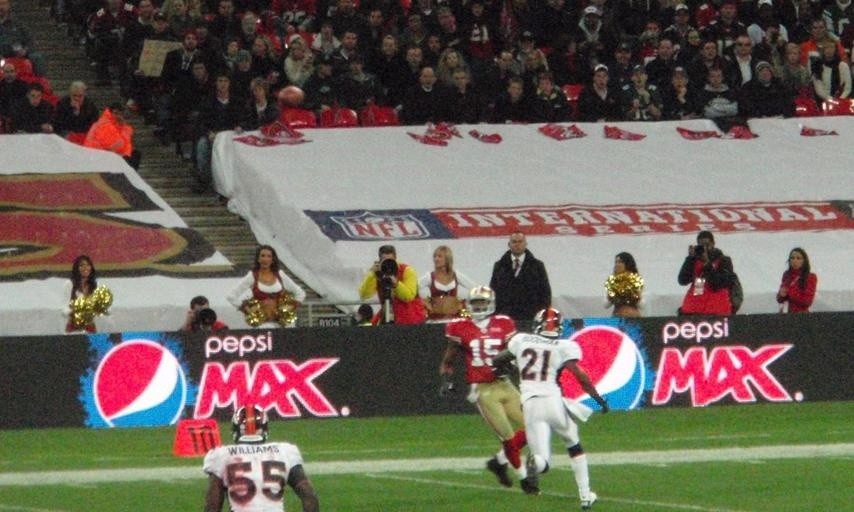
[0,59,87,146]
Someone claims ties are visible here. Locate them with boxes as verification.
[512,259,520,277]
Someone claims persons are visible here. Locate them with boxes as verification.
[225,244,306,329]
[61,254,113,335]
[438,285,540,492]
[489,231,551,320]
[600,252,647,317]
[201,404,319,511]
[493,308,608,510]
[358,245,426,324]
[176,294,230,331]
[775,247,818,312]
[418,246,476,320]
[675,229,744,318]
[0,1,853,185]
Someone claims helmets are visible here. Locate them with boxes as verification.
[231,405,270,444]
[467,286,499,315]
[532,306,563,340]
[486,311,517,349]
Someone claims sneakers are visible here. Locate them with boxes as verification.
[581,491,598,509]
[521,476,541,495]
[527,453,537,473]
[486,457,512,487]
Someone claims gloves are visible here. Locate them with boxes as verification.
[438,372,461,399]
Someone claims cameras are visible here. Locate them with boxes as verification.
[694,244,704,258]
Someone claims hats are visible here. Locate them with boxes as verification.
[519,31,534,40]
[670,0,854,79]
[594,64,609,73]
[632,64,647,74]
[617,41,632,50]
[549,26,587,49]
[584,6,603,17]
